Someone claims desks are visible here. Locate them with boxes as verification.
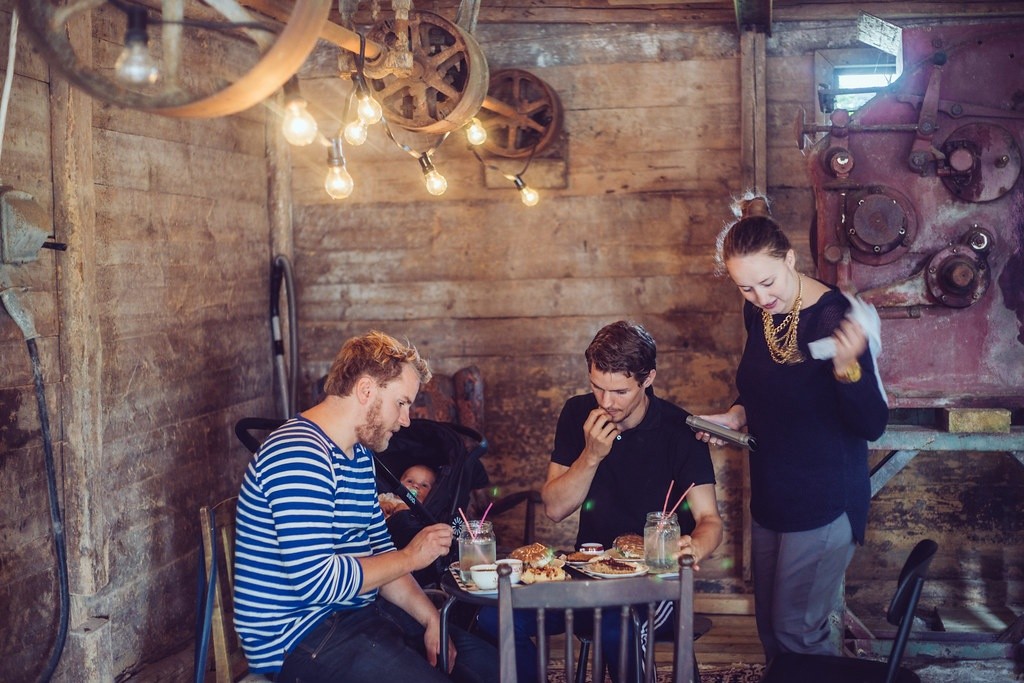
[440,552,683,683]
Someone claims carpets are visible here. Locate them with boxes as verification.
[543,653,1024,683]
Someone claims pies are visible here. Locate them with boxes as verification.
[567,551,636,574]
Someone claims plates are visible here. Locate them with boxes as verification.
[449,560,521,596]
[566,548,650,578]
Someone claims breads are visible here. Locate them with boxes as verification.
[506,543,566,584]
[613,535,644,559]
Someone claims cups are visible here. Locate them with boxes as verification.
[469,558,523,590]
[579,543,604,555]
[642,511,681,575]
[460,520,498,581]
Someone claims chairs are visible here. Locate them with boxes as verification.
[200,497,271,683]
[760,538,940,683]
[493,565,696,683]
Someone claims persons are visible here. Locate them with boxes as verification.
[233,330,500,683]
[477,320,723,683]
[378,464,440,521]
[695,215,890,668]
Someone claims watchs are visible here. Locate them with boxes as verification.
[832,362,861,384]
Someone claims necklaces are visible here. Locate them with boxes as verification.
[761,273,808,366]
